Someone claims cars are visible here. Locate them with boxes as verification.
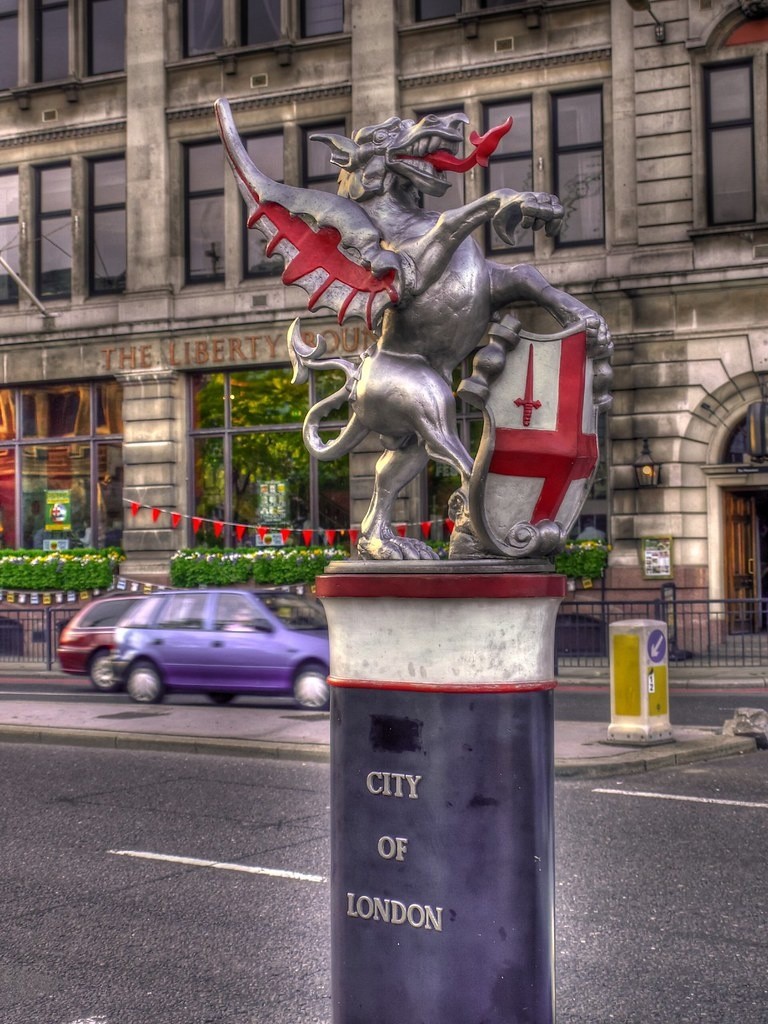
[56,591,169,694]
[110,589,342,711]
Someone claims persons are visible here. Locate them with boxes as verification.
[220,602,259,634]
